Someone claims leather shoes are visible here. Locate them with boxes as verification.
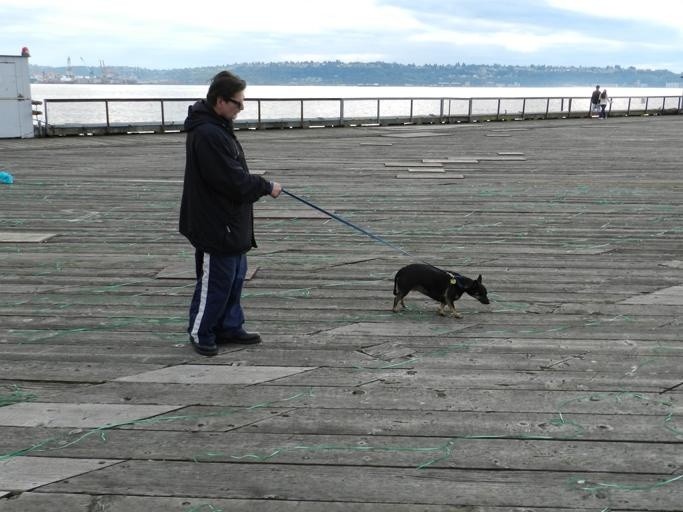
[190,335,219,357]
[223,330,263,345]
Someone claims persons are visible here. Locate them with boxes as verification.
[589,85,601,119]
[596,90,614,119]
[178,71,281,356]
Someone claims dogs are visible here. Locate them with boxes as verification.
[393,264,490,318]
